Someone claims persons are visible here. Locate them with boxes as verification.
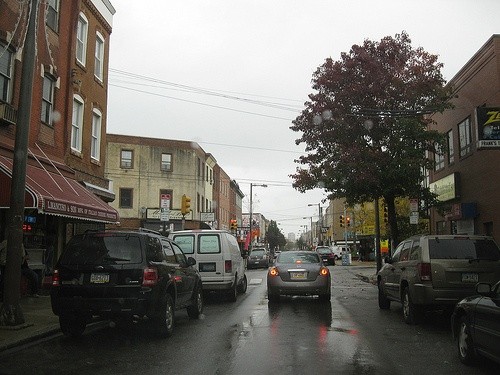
[0,239,38,300]
[359,244,373,261]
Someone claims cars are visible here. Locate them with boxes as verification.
[451,280,500,368]
[245,248,271,269]
[274,251,281,259]
[266,251,332,303]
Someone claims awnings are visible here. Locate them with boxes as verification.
[0,155,119,224]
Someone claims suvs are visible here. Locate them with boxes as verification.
[377,235,500,325]
[330,245,351,260]
[166,229,248,301]
[50,226,204,339]
[313,246,335,266]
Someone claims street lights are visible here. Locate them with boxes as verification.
[300,224,307,249]
[249,183,268,254]
[303,217,313,249]
[308,203,321,246]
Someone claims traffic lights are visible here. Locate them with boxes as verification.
[346,216,350,227]
[233,220,238,229]
[230,220,234,230]
[180,195,191,214]
[384,206,388,222]
[339,215,345,228]
